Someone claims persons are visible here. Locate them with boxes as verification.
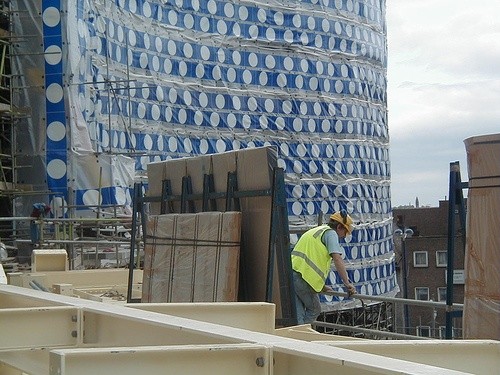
[291,209,356,327]
[29,203,51,246]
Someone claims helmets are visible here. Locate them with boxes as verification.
[329,211,354,233]
